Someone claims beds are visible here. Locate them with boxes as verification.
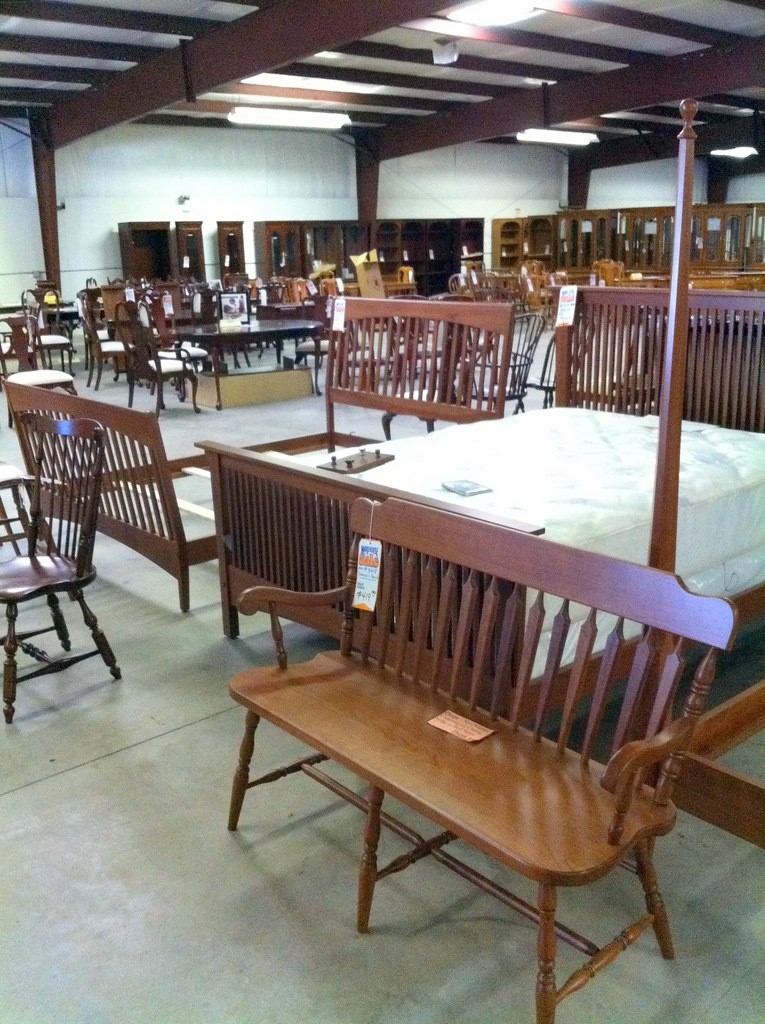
[195,286,765,727]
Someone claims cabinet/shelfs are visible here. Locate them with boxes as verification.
[116,202,764,289]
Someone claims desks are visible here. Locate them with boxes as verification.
[169,319,322,411]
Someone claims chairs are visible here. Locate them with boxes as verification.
[0,258,630,427]
[0,414,122,723]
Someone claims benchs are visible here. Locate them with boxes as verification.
[226,495,740,1024]
